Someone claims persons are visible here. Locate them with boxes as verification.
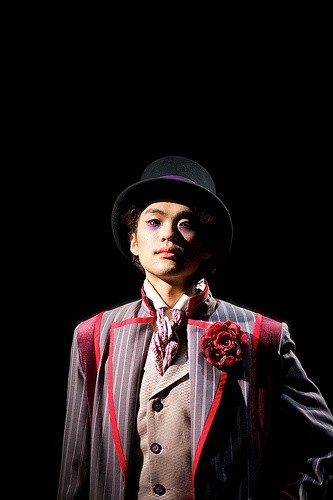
[58,152,333,500]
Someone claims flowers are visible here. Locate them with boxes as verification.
[200,321,249,368]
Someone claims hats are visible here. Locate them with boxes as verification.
[110,156,233,278]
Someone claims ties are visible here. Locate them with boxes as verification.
[152,309,185,378]
